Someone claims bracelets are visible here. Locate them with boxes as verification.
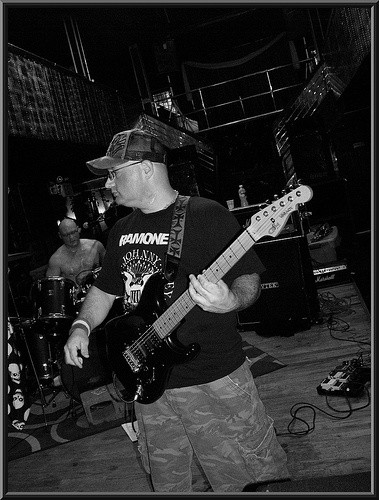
[68,318,92,336]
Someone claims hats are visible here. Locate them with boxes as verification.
[86,128,170,176]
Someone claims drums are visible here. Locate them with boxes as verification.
[26,275,81,333]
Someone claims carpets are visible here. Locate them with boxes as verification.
[6,338,289,464]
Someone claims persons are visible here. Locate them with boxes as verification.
[65,128,284,493]
[46,219,106,288]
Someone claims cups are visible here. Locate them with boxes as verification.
[226,199,234,210]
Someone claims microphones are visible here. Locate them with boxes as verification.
[83,219,105,229]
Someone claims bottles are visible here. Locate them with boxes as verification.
[238,185,248,207]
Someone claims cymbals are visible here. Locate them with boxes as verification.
[7,252,33,261]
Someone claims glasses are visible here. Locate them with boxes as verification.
[70,228,79,234]
[107,160,141,181]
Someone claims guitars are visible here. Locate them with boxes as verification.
[91,179,313,405]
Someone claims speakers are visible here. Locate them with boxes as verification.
[234,235,318,332]
